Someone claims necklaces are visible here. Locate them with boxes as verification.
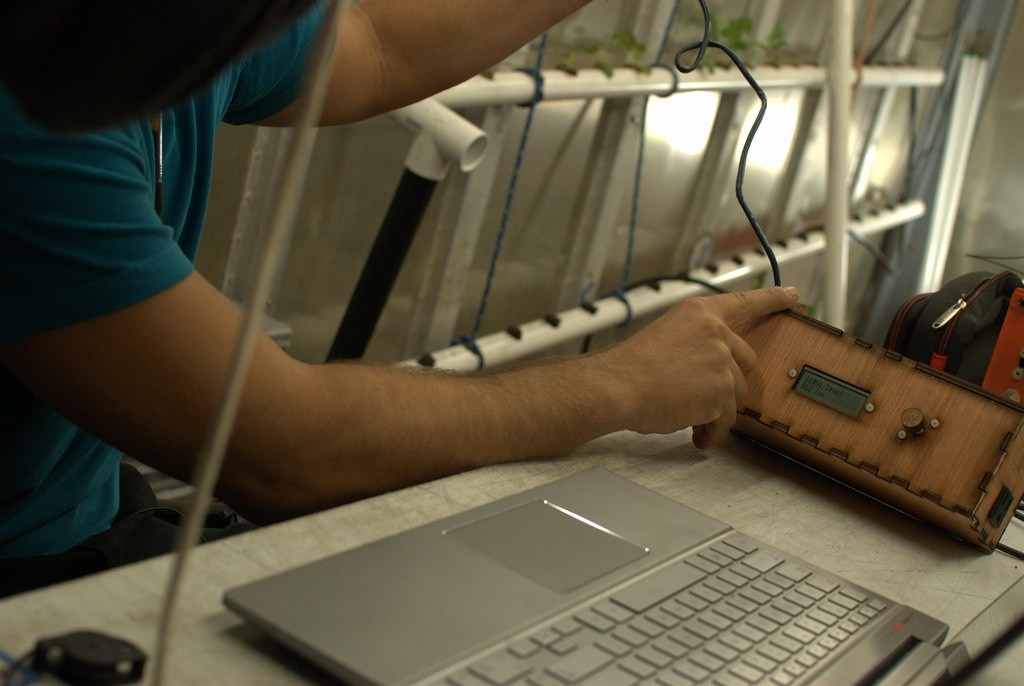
[156,112,163,214]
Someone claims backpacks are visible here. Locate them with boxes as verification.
[885,271,1023,389]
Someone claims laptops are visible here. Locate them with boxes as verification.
[217,466,1024,686]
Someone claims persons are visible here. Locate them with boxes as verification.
[0,0,800,600]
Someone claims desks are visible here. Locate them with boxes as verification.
[0,422,1022,684]
